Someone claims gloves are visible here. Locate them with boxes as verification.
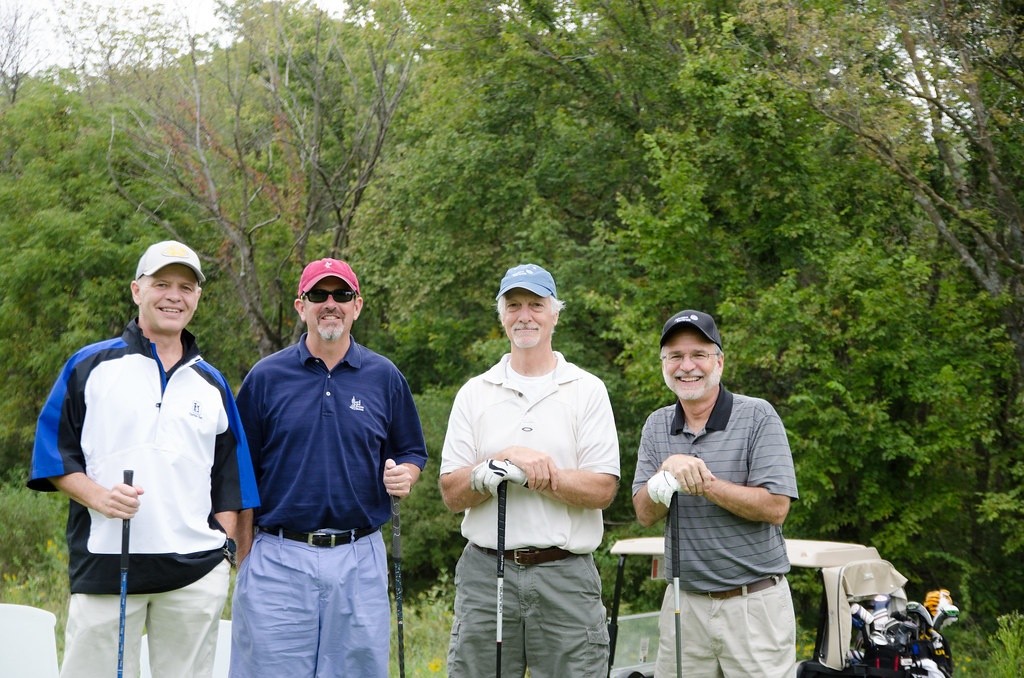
[646,469,687,507]
[469,459,529,498]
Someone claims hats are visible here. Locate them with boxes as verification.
[296,258,360,298]
[493,263,557,301]
[657,310,722,349]
[134,239,207,284]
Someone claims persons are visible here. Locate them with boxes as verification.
[26,241,262,678]
[228,259,430,678]
[632,311,799,678]
[438,264,621,678]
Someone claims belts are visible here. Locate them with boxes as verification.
[695,573,785,599]
[260,524,384,548]
[472,544,573,565]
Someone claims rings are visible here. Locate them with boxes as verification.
[396,482,399,489]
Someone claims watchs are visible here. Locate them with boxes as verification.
[223,537,237,554]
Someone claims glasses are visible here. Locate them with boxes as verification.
[302,289,358,303]
[661,350,721,363]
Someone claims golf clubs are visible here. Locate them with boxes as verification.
[495,480,507,678]
[117,469,134,678]
[670,492,682,678]
[868,601,934,670]
[391,495,406,678]
